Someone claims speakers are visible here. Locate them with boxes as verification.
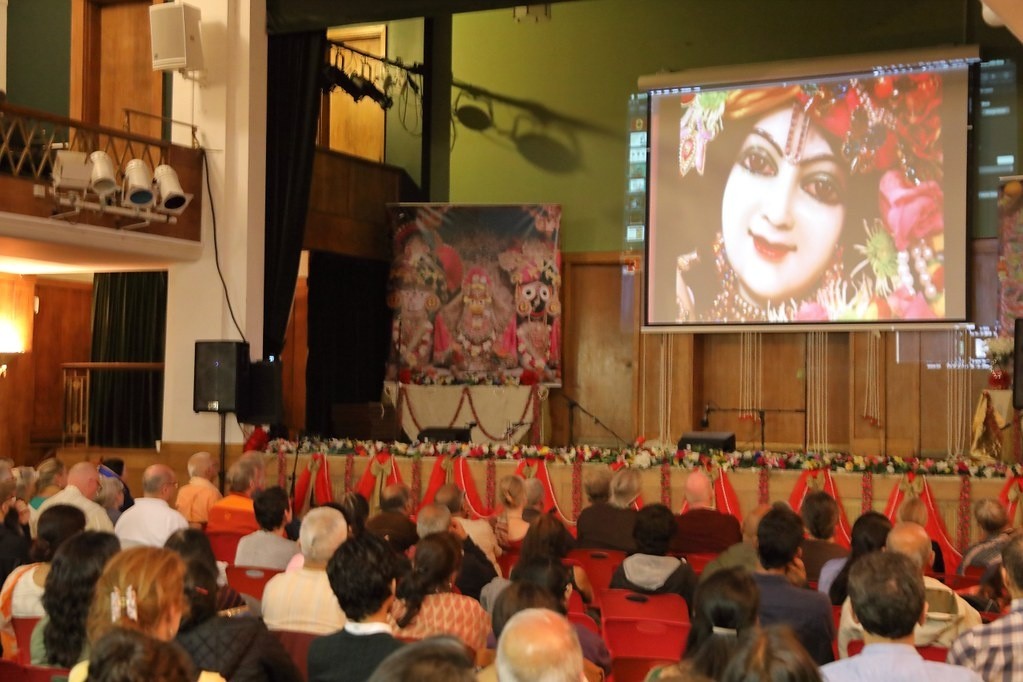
[1013,318,1023,409]
[192,339,284,426]
[418,426,471,444]
[677,430,736,467]
[149,2,203,71]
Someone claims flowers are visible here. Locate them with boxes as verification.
[988,336,1014,369]
[386,367,539,387]
[269,434,1022,478]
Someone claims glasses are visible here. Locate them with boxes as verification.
[96,479,103,493]
[165,481,179,491]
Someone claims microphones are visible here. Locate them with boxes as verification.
[466,423,477,426]
[701,403,710,428]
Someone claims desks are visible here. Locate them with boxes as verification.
[377,381,548,448]
[974,388,1017,462]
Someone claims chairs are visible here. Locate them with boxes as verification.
[5,530,951,681]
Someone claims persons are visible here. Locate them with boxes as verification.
[0,451,1023,682]
[676,92,878,321]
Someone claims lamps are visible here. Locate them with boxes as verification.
[48,150,194,215]
[321,45,392,110]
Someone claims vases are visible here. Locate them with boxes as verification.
[988,366,1008,389]
